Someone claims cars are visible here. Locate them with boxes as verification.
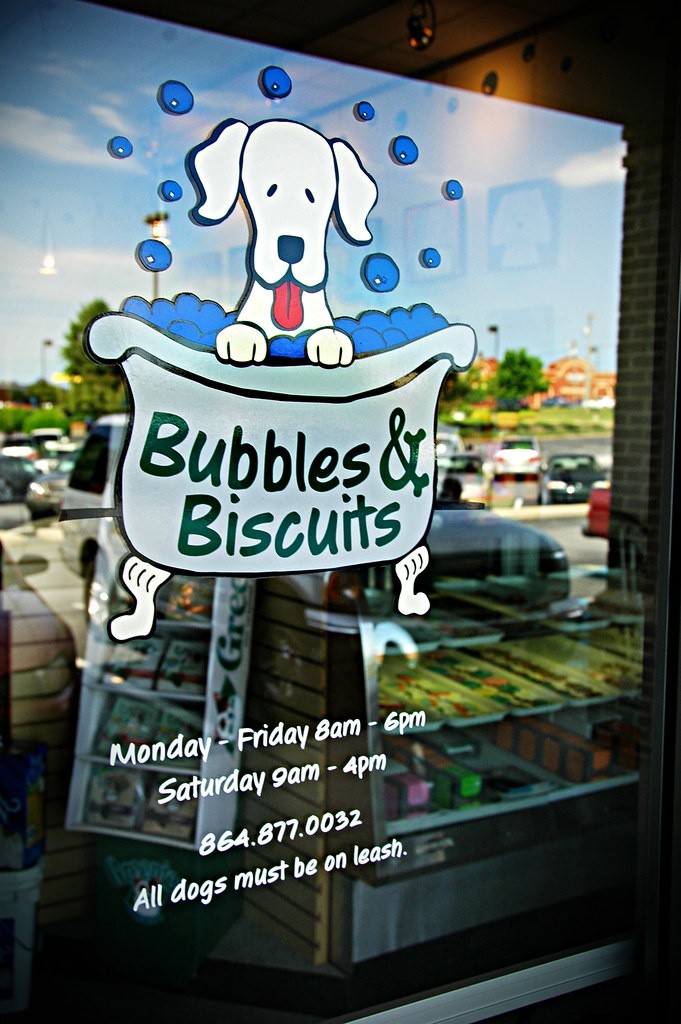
[541,454,609,506]
[0,428,84,519]
[58,415,572,641]
[434,426,543,507]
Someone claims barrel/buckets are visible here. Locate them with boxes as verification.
[0,739,48,871]
